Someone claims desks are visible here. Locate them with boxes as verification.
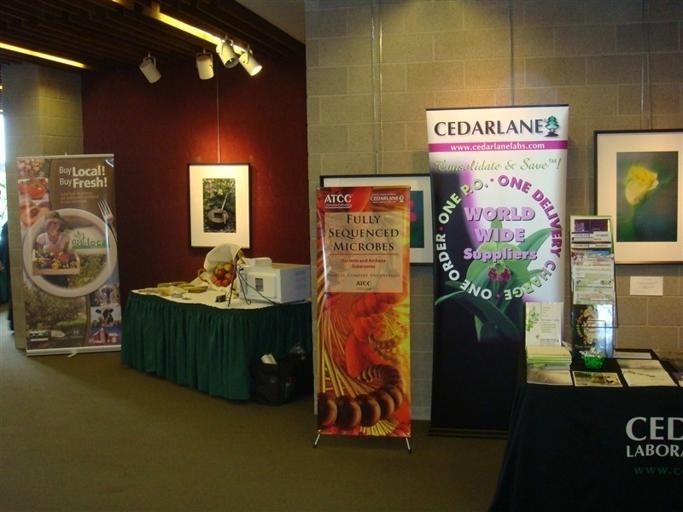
[489,347,683,512]
[120,282,311,403]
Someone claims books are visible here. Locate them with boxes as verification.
[523,214,682,391]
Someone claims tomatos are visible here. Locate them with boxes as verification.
[19,179,48,199]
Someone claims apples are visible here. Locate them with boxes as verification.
[211,263,236,287]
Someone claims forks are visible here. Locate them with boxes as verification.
[97,200,118,243]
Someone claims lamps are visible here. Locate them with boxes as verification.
[139,48,161,84]
[230,42,262,77]
[195,44,214,81]
[215,38,239,70]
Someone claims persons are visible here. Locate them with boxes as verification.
[35,212,73,288]
[1,222,14,332]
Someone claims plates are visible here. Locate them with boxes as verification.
[22,206,117,296]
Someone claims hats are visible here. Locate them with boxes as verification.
[45,211,65,229]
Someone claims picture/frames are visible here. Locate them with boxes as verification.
[318,172,436,267]
[592,127,683,265]
[187,162,251,251]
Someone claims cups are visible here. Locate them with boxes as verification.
[582,355,606,370]
